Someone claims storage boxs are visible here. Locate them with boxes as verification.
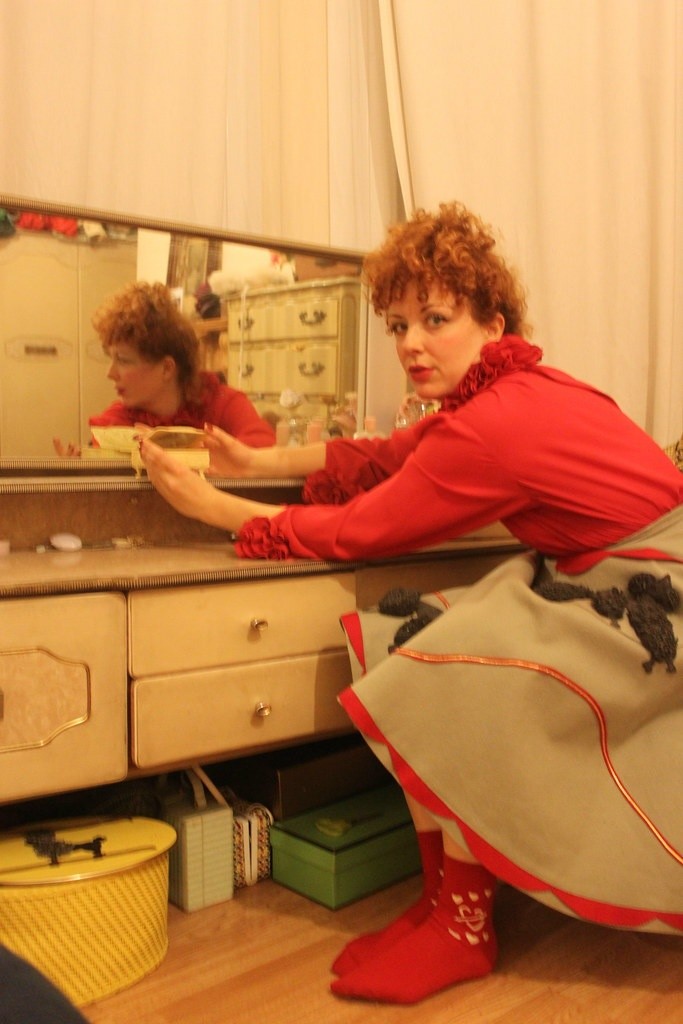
[269,782,421,911]
[161,798,236,912]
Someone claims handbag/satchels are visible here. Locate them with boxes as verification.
[156,765,274,914]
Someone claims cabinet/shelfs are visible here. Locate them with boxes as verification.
[0,553,520,806]
[1,229,139,459]
[227,286,360,425]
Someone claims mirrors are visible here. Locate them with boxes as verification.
[0,196,369,494]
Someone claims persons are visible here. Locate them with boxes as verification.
[137,201,682,1004]
[89,280,272,460]
